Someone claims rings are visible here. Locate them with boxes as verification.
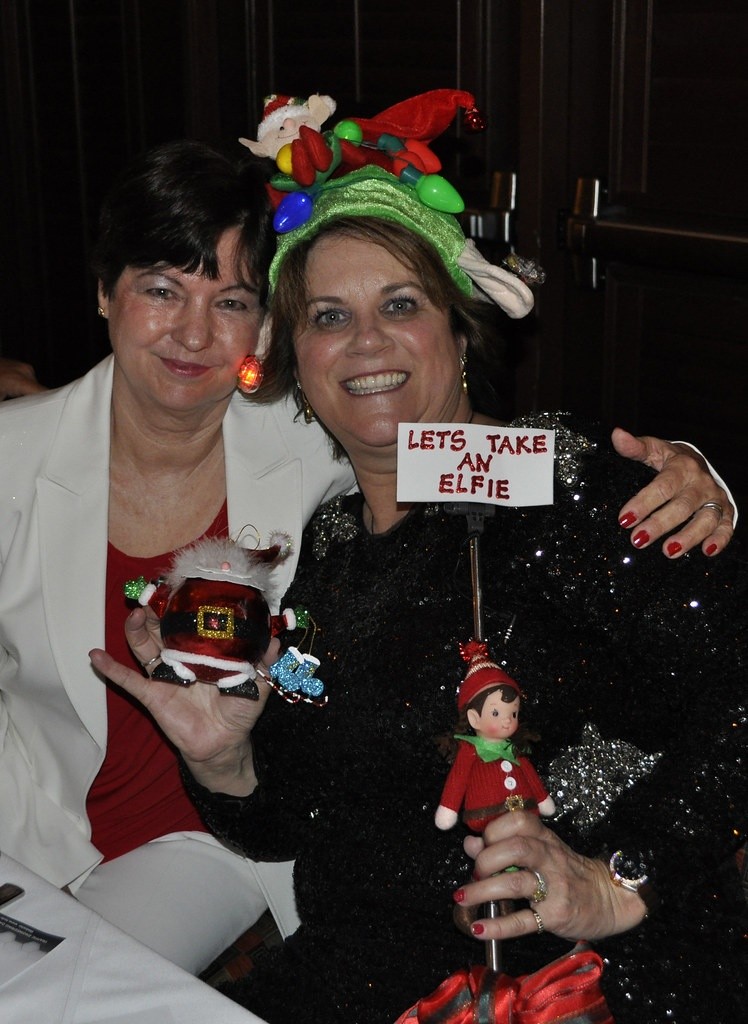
[699,502,727,515]
[142,650,161,669]
[523,907,546,936]
[524,867,552,904]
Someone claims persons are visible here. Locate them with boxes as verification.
[85,169,744,1022]
[0,84,747,1024]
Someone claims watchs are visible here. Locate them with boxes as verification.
[591,842,665,917]
